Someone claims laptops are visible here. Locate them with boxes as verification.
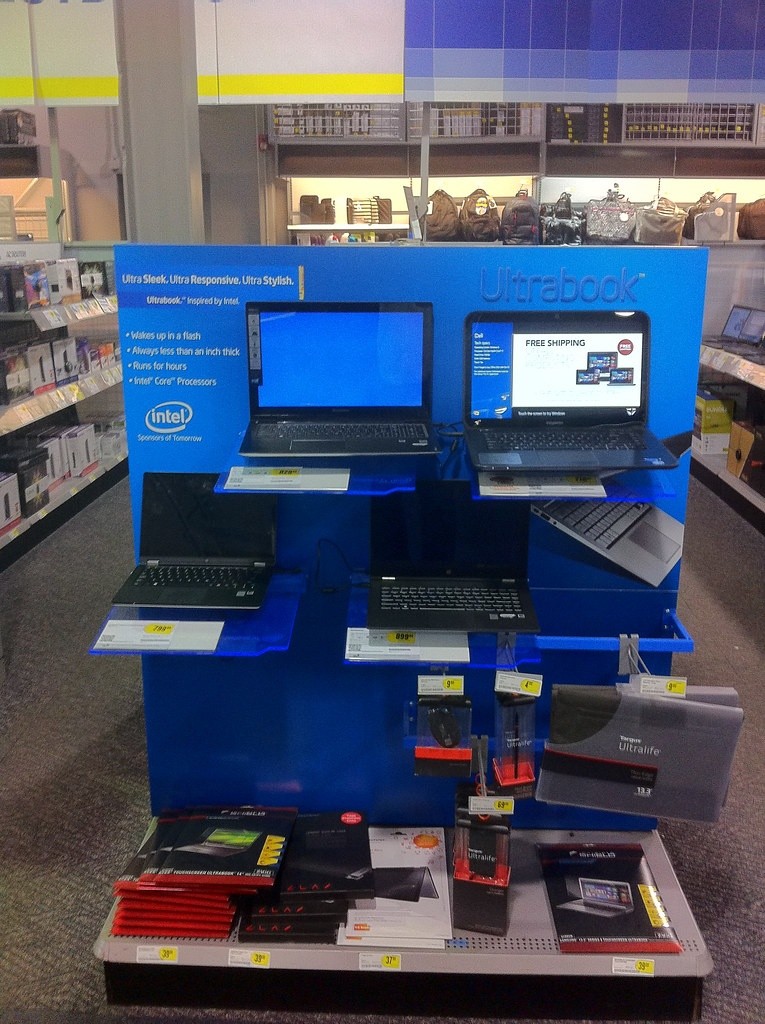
[172,827,263,858]
[237,302,442,457]
[531,495,684,587]
[464,310,680,473]
[701,305,765,365]
[368,480,543,632]
[607,368,634,385]
[110,472,276,609]
[556,877,634,919]
[372,867,440,902]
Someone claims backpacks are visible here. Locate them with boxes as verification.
[501,188,540,245]
[459,188,500,241]
[418,189,458,241]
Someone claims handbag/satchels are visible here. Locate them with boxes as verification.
[681,193,735,239]
[586,193,636,241]
[541,193,584,245]
[637,198,688,246]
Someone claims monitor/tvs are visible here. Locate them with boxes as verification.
[587,352,618,373]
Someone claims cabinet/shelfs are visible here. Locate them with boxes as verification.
[690,336,765,537]
[0,241,129,573]
[86,428,713,1019]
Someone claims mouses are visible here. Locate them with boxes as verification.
[427,708,462,748]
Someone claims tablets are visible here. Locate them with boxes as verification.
[576,369,600,385]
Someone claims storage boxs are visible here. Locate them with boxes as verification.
[1,110,36,144]
[691,382,765,498]
[0,258,127,535]
